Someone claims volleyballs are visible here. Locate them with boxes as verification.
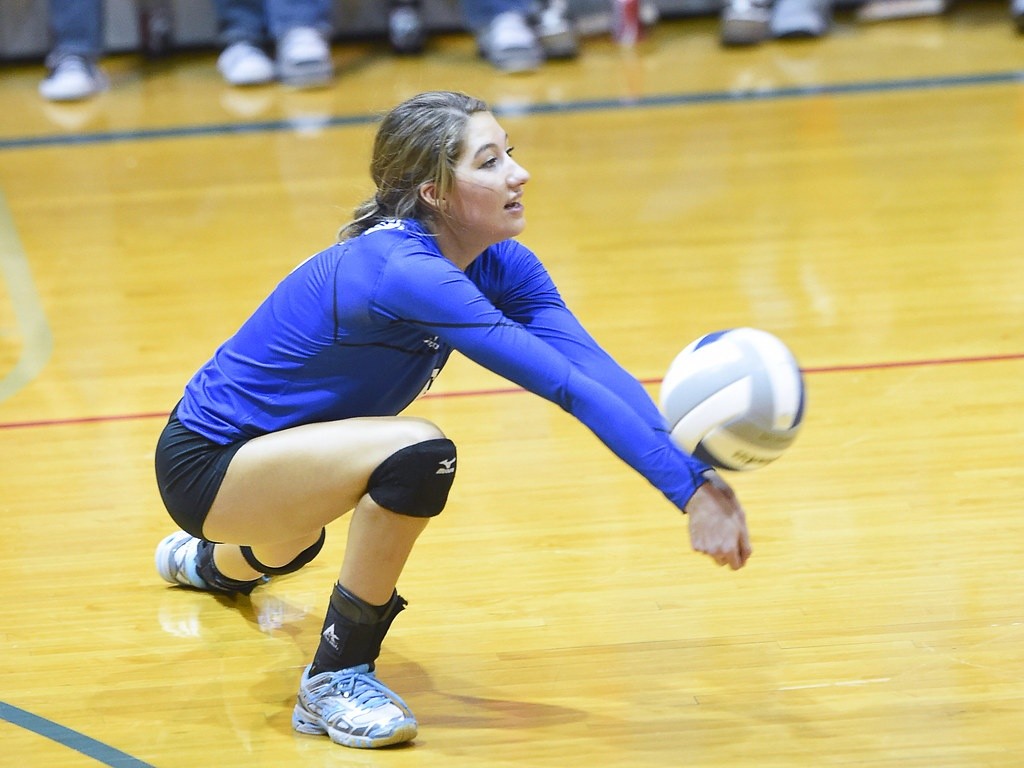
[659,327,807,471]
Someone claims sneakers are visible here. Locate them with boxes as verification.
[155,529,207,589]
[291,661,419,748]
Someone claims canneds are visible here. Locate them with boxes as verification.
[615,0,641,44]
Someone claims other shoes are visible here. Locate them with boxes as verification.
[387,12,428,53]
[478,10,540,72]
[535,15,578,59]
[38,54,99,100]
[214,42,276,86]
[275,24,329,73]
[717,4,769,43]
[771,1,829,38]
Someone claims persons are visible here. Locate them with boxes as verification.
[38,0,1024,102]
[154,91,754,749]
